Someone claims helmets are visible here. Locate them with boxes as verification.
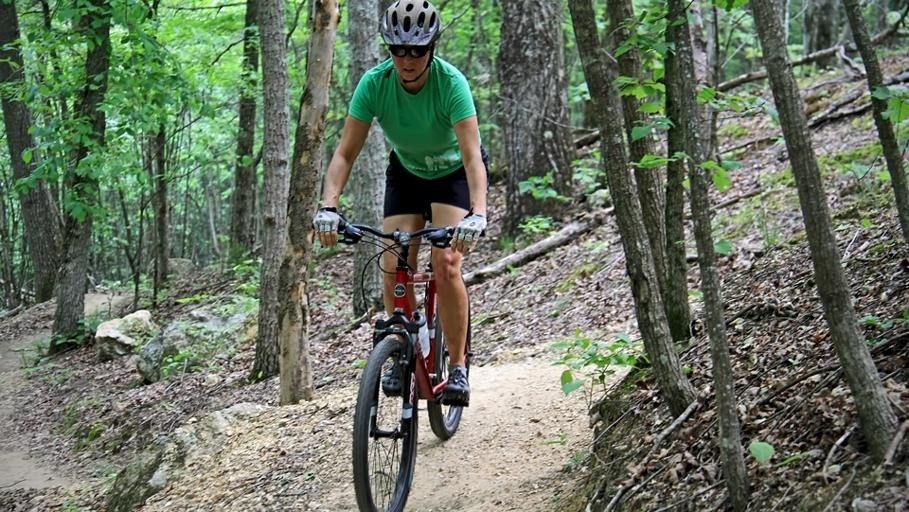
[381,0,439,46]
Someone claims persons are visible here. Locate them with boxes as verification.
[310,0,490,408]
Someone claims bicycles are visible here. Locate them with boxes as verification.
[310,213,473,510]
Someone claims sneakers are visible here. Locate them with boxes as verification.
[382,361,406,395]
[443,369,470,400]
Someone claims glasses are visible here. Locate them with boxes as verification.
[388,37,432,58]
